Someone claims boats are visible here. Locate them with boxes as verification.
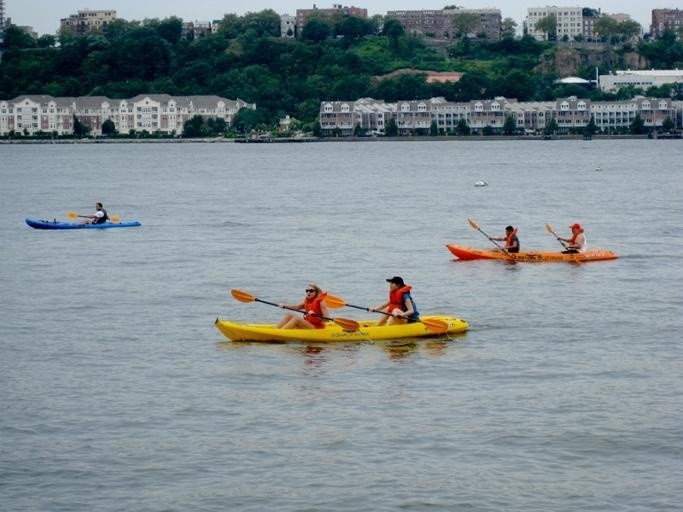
[26,215,142,230]
[210,312,472,344]
[442,241,621,264]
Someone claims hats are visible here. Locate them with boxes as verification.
[386,276,406,287]
[568,223,581,231]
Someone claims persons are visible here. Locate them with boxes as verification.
[364,276,420,326]
[556,224,586,253]
[274,284,330,329]
[489,225,520,254]
[81,202,107,225]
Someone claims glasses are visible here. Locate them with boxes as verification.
[305,289,316,293]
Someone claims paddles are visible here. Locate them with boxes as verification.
[230,288,360,332]
[467,216,516,264]
[68,212,120,222]
[546,222,582,265]
[324,293,448,334]
